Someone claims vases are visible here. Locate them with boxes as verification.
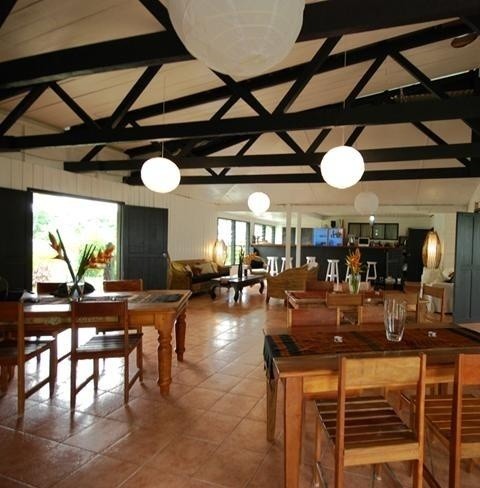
[349,275,361,295]
[66,277,85,301]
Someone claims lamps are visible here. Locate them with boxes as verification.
[167,0,305,77]
[141,71,181,193]
[320,49,365,190]
[422,231,441,283]
[212,240,227,265]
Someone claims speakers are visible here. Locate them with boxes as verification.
[330,220,336,227]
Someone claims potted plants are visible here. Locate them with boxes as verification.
[238,245,244,277]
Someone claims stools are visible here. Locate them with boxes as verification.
[266,256,378,287]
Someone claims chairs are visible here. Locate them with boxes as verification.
[0,279,143,414]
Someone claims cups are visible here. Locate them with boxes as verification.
[384,298,405,343]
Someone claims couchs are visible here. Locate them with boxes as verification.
[266,264,320,306]
[168,259,232,297]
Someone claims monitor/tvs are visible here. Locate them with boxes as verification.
[358,237,370,247]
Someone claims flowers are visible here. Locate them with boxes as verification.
[48,229,115,300]
[345,248,362,293]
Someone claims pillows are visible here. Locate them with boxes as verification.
[172,262,219,277]
